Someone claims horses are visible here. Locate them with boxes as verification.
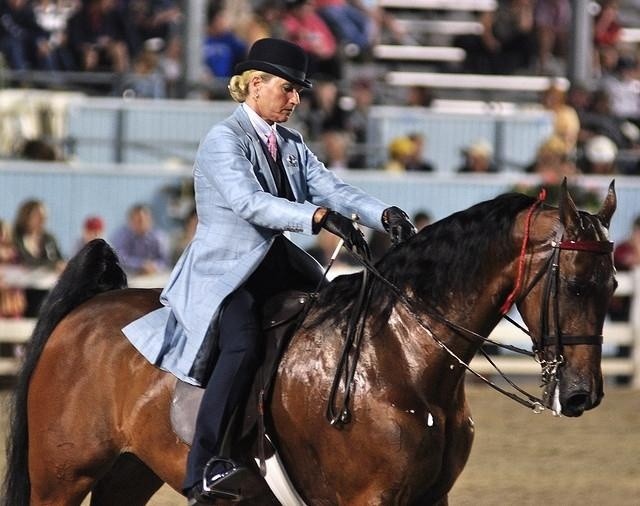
[0,175,619,506]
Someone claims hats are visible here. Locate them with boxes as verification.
[233,38,312,88]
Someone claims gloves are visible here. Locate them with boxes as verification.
[380,206,417,243]
[318,206,373,258]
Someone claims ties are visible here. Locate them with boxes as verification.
[266,133,277,161]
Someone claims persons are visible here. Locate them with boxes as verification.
[1,0,638,387]
[121,36,416,506]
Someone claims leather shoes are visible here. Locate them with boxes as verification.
[188,466,260,506]
